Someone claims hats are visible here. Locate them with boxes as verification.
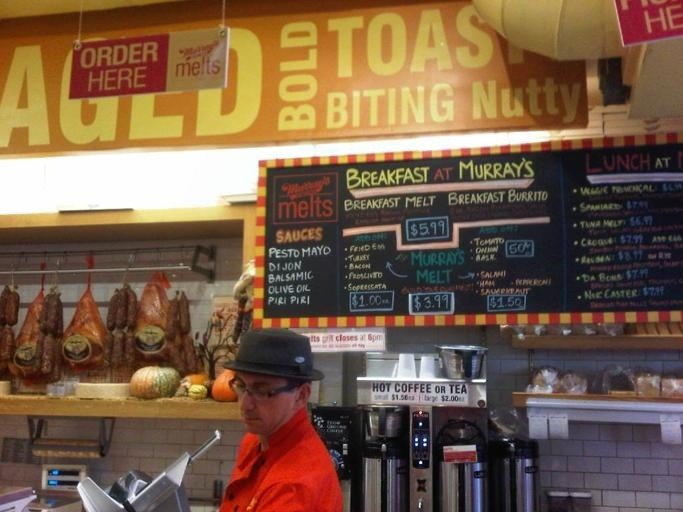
[221,325,325,383]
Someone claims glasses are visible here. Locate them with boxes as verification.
[228,376,299,400]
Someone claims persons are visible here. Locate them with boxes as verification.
[219,328,343,512]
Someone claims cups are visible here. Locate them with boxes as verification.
[393,353,435,378]
[546,490,592,512]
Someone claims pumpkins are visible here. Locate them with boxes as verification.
[131,364,181,399]
[211,366,237,402]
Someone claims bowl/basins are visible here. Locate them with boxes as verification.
[436,344,488,378]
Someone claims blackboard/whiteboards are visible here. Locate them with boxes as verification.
[252,133,682,331]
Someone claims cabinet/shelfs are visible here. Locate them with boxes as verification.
[0,199,255,424]
[512,330,683,409]
